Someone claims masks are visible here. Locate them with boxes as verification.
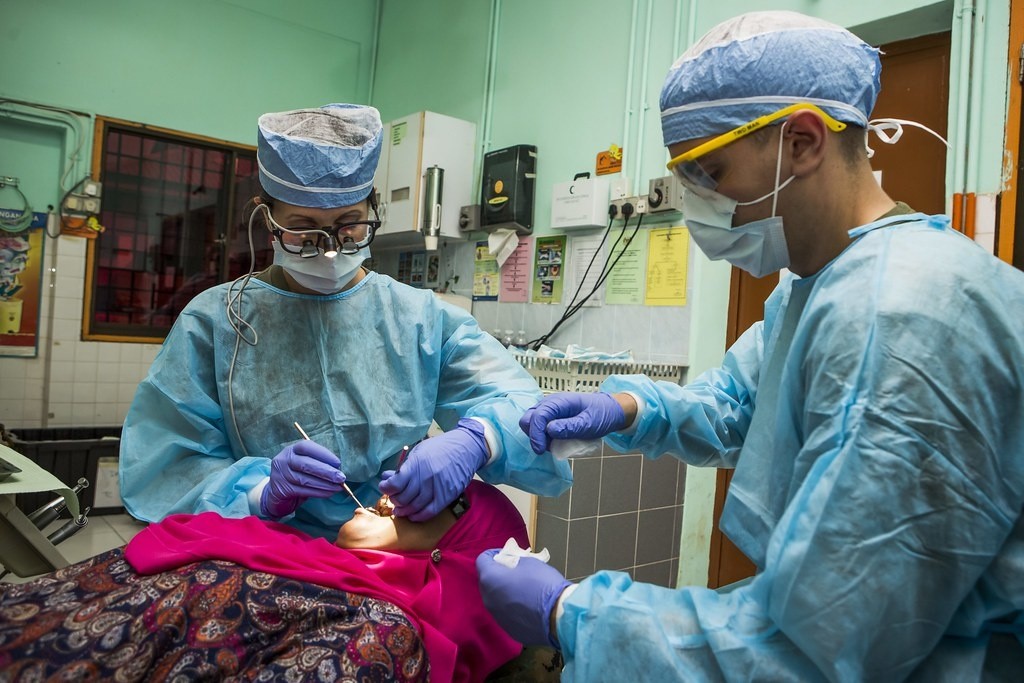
[681,120,798,279]
[270,239,372,295]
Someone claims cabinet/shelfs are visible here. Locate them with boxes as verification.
[365,109,477,254]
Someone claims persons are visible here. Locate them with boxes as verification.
[474,9,1024,683]
[0,477,529,683]
[118,99,573,542]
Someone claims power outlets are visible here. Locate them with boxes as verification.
[64,179,102,216]
[609,195,651,220]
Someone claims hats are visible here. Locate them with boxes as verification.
[659,10,884,148]
[257,103,383,209]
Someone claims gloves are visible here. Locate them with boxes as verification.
[260,440,346,520]
[378,417,490,523]
[517,391,625,455]
[475,548,572,652]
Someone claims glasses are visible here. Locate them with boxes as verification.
[665,100,848,201]
[266,188,381,259]
[449,492,469,520]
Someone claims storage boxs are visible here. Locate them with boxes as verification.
[3,425,132,519]
